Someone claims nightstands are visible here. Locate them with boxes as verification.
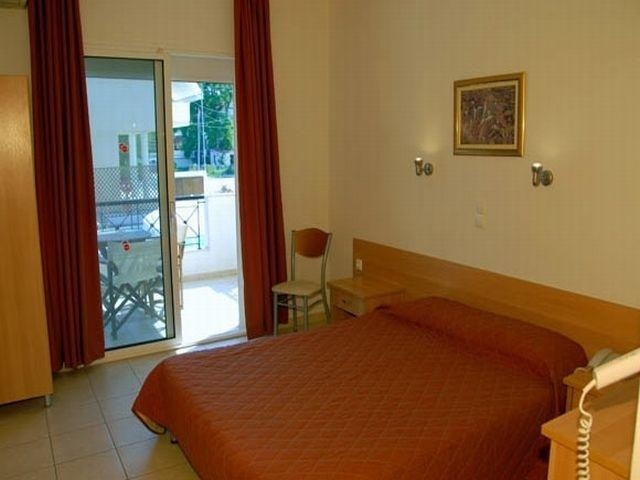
[564,368,623,411]
[327,272,404,326]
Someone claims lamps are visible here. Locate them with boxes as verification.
[414,158,433,176]
[531,162,553,186]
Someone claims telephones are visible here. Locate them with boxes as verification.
[587,347,621,368]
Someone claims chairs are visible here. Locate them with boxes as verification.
[93,205,186,339]
[272,227,333,334]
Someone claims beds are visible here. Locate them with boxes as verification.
[132,238,639,480]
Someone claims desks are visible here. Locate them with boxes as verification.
[541,378,639,480]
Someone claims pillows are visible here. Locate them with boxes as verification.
[376,296,588,419]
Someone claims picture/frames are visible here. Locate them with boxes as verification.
[453,72,526,157]
